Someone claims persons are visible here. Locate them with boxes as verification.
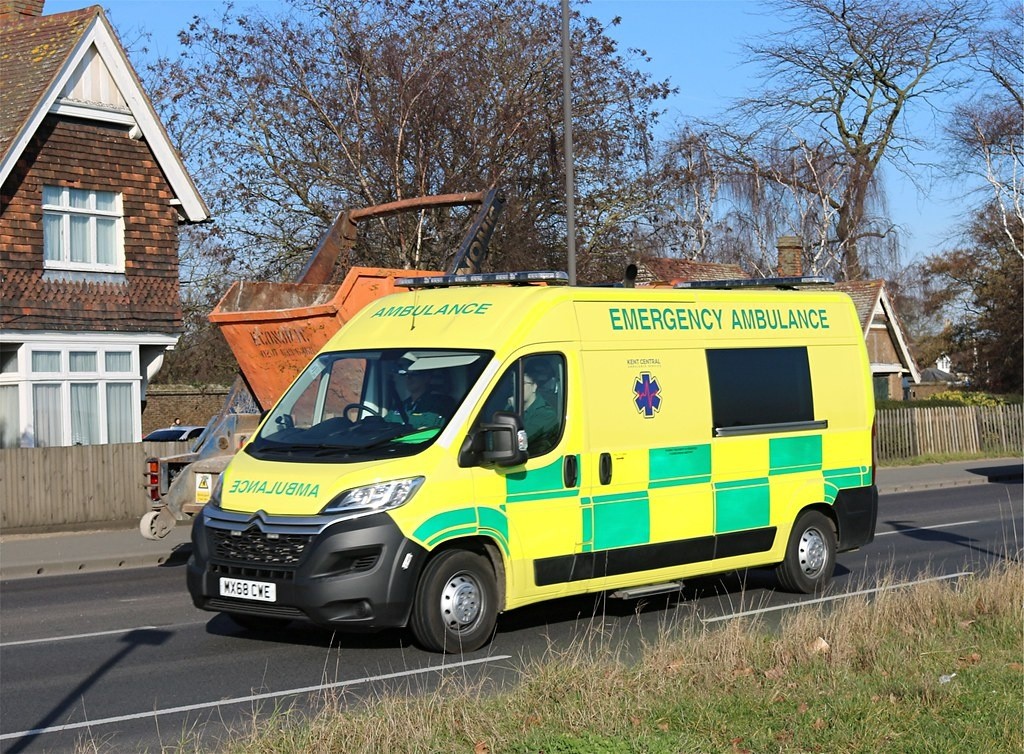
[383,363,455,429]
[488,363,560,455]
[170,418,181,428]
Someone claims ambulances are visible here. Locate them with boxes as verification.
[185,271,880,654]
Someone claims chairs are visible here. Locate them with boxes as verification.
[535,374,558,415]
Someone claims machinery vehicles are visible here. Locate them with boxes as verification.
[140,187,689,566]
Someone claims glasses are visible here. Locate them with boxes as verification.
[403,371,426,378]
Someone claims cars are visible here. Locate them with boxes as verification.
[143,426,204,500]
